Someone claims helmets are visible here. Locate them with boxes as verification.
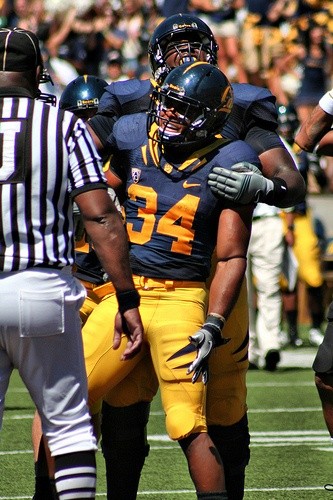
[60,76,108,109]
[146,63,234,146]
[149,14,216,86]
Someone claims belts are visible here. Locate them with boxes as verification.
[92,274,204,299]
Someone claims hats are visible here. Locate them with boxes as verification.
[0,27,43,73]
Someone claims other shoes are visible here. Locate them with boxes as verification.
[264,350,280,370]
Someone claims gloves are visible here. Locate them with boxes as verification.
[186,315,226,385]
[207,163,287,207]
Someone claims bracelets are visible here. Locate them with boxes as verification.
[116,289,140,308]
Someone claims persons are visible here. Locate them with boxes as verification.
[0,0,333,191]
[295,89,333,437]
[33,75,108,457]
[36,60,264,500]
[0,27,143,500]
[243,136,299,369]
[97,13,306,500]
[280,211,327,348]
